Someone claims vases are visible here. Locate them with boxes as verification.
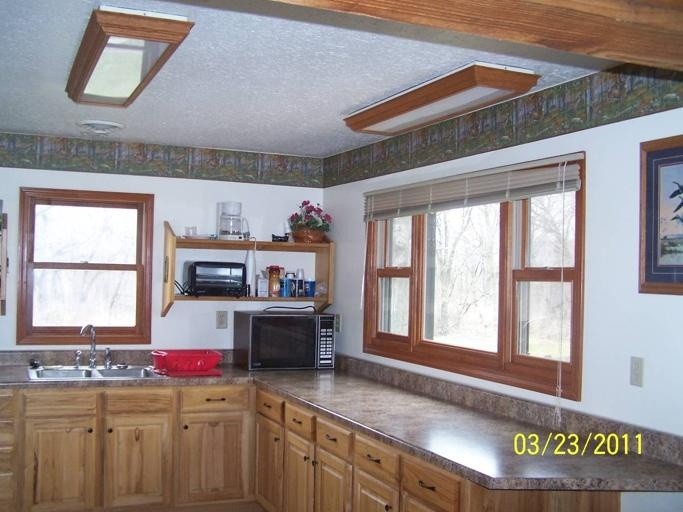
[293,229,325,243]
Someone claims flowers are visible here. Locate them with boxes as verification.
[288,201,332,232]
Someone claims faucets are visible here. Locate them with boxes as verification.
[80,323,97,368]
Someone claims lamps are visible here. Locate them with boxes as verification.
[64,4,195,108]
[342,61,542,136]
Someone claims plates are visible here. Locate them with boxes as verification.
[184,234,208,240]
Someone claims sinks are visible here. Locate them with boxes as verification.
[97,366,165,378]
[36,367,98,379]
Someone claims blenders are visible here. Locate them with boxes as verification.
[215,200,250,243]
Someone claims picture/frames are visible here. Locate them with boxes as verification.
[639,134,683,295]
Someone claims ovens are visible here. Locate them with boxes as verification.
[182,260,246,297]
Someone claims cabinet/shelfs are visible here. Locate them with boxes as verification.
[162,221,336,314]
[285,402,354,512]
[354,433,463,512]
[177,385,250,507]
[255,387,284,512]
[20,387,175,512]
[0,389,22,512]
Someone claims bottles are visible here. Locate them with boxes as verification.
[256,264,316,298]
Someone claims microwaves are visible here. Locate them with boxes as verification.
[232,309,335,370]
